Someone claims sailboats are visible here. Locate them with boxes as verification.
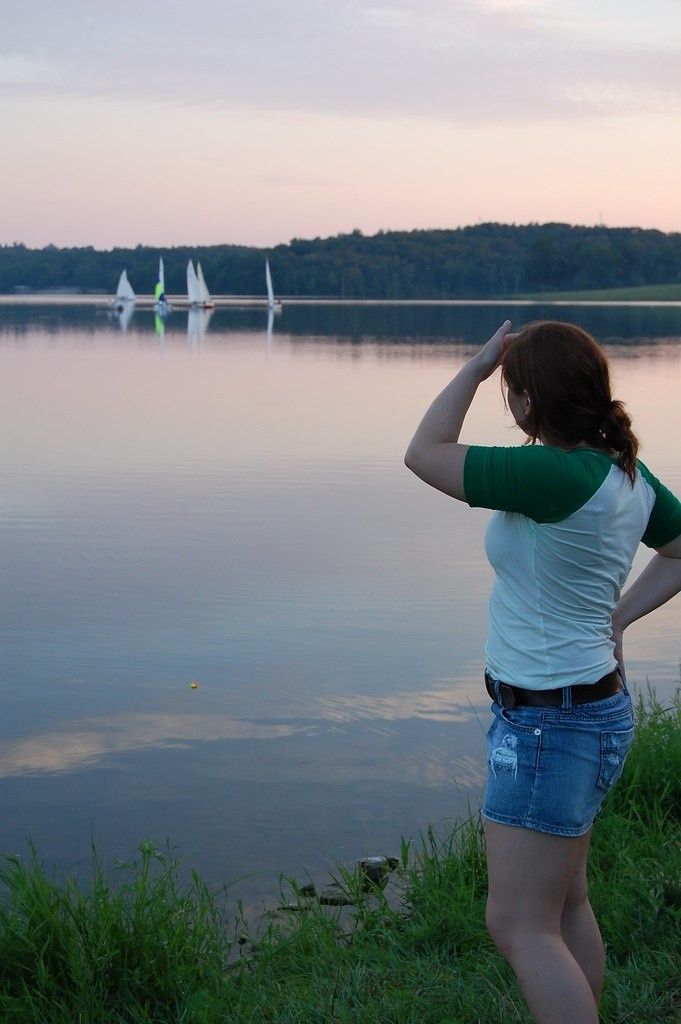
[153,257,173,314]
[187,311,213,348]
[155,315,169,342]
[117,313,132,333]
[113,270,136,313]
[265,258,282,309]
[267,310,281,344]
[184,257,216,310]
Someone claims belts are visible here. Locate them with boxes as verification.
[483,670,623,711]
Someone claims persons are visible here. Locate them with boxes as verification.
[403,320,681,1024]
